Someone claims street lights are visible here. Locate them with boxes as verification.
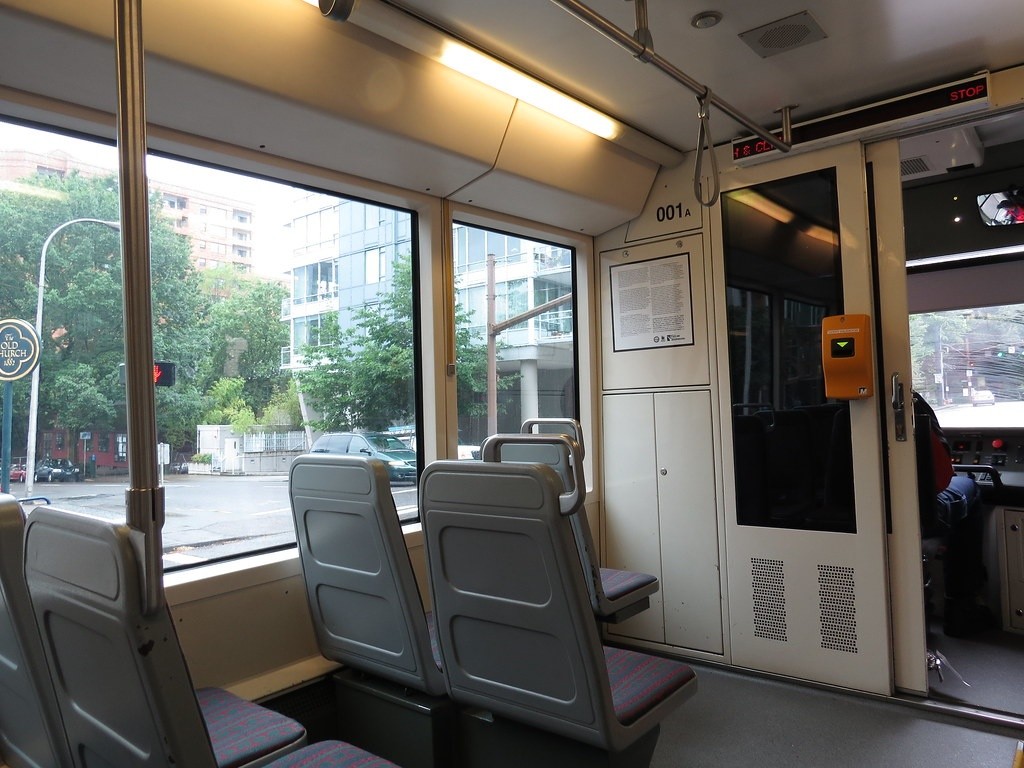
[20,218,124,507]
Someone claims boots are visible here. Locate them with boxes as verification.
[942,595,995,637]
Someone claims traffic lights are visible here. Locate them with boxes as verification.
[154,361,178,392]
[998,352,1007,357]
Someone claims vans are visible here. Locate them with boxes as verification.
[310,433,417,485]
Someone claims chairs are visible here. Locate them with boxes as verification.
[478,431,661,646]
[418,460,702,767]
[21,508,400,767]
[289,453,451,768]
[0,491,309,768]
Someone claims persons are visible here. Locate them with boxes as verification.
[911,390,981,639]
[997,199,1024,222]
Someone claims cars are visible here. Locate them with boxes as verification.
[0,463,27,482]
[34,458,82,482]
[972,390,996,406]
